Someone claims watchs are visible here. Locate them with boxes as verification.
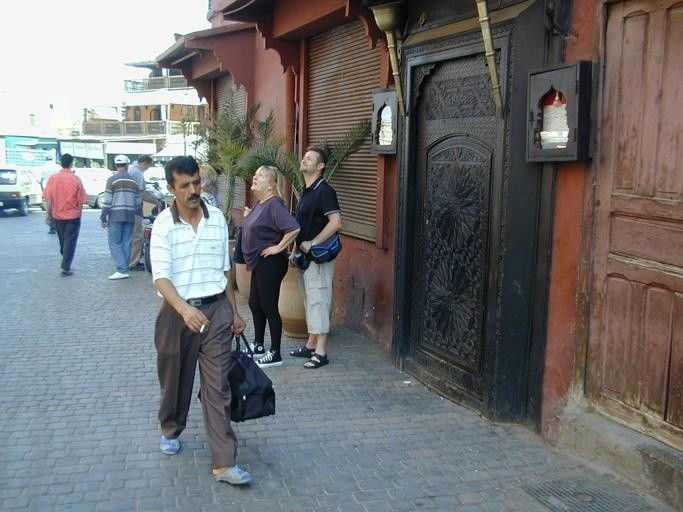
[311,240,316,247]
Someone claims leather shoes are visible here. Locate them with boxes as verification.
[128,262,146,272]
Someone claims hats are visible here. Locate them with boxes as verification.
[114,155,130,164]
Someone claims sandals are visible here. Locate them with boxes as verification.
[303,351,329,370]
[290,345,315,358]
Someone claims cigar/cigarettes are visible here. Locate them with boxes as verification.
[200,325,206,333]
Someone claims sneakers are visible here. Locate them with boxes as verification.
[214,464,252,485]
[241,343,265,358]
[108,271,130,280]
[159,434,181,455]
[254,350,283,369]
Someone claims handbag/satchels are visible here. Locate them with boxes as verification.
[197,329,277,424]
[232,224,248,265]
[292,250,313,270]
[310,229,342,264]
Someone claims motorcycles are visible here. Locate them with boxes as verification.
[140,182,170,275]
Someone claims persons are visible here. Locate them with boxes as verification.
[240,164,301,367]
[45,155,89,275]
[33,155,64,231]
[148,157,255,487]
[288,149,339,368]
[126,157,159,270]
[100,156,142,279]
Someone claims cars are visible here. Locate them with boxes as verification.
[0,164,117,220]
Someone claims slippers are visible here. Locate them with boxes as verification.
[60,271,74,277]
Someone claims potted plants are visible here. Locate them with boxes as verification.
[230,120,376,339]
[190,106,290,309]
